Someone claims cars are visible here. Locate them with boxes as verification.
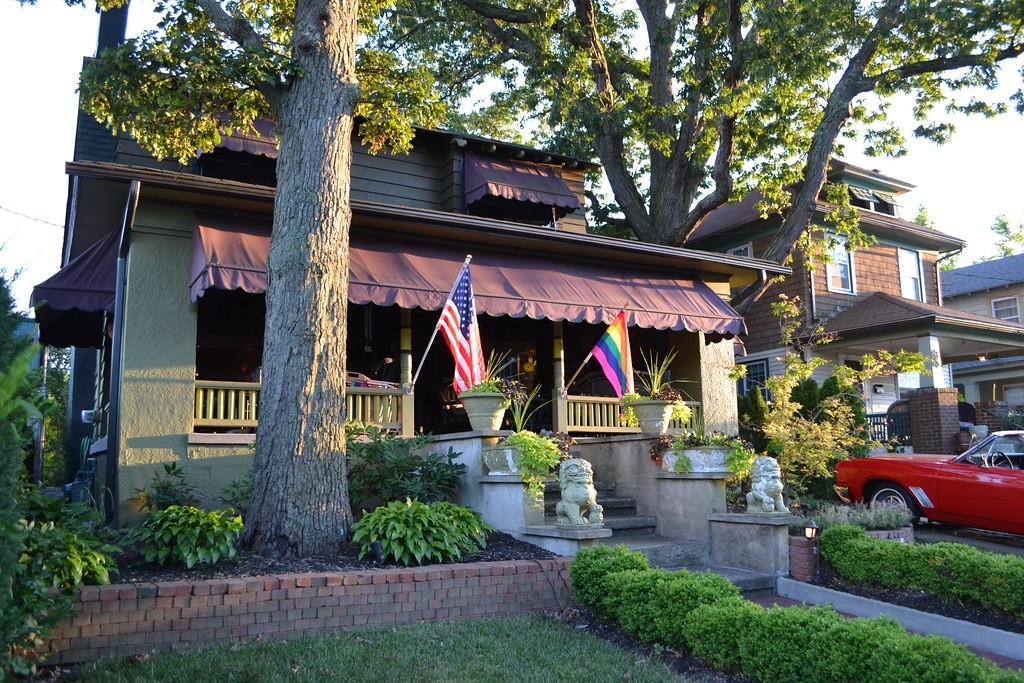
[837,431,1024,535]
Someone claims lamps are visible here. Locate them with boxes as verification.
[802,517,818,540]
[872,384,884,393]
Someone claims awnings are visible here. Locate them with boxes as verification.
[197,107,281,161]
[464,150,580,225]
[28,221,122,349]
[188,209,749,345]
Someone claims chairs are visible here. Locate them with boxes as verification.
[958,400,976,430]
[886,400,909,442]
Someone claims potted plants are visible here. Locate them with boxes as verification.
[481,382,568,510]
[457,345,529,432]
[617,346,701,435]
[649,428,755,479]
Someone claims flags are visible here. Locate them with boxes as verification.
[436,262,486,405]
[591,310,635,417]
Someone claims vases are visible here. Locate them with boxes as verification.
[956,425,988,444]
